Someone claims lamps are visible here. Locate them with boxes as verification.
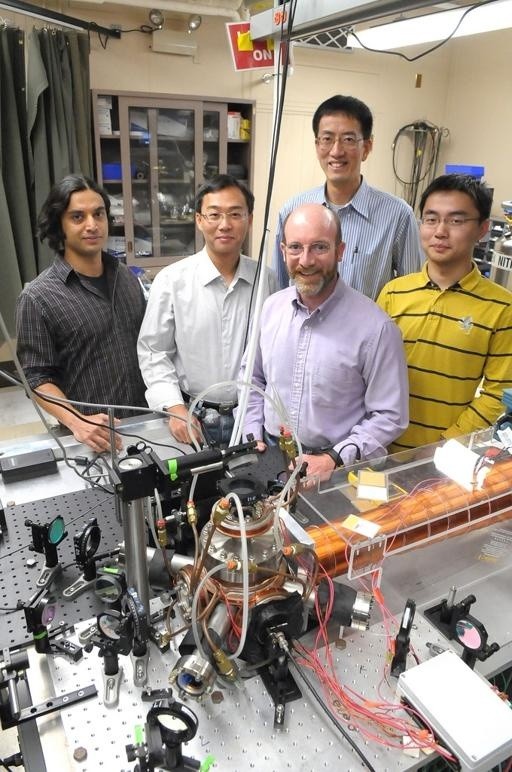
[347,0,512,53]
[149,9,202,34]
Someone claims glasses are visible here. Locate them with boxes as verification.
[285,241,337,254]
[420,215,481,227]
[316,136,365,148]
[200,210,249,223]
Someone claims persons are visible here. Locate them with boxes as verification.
[272,95,428,304]
[136,175,276,452]
[241,203,410,490]
[374,174,512,469]
[16,174,149,454]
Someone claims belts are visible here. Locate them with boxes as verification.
[267,433,299,453]
[181,390,238,415]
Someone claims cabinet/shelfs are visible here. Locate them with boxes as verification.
[92,90,256,279]
